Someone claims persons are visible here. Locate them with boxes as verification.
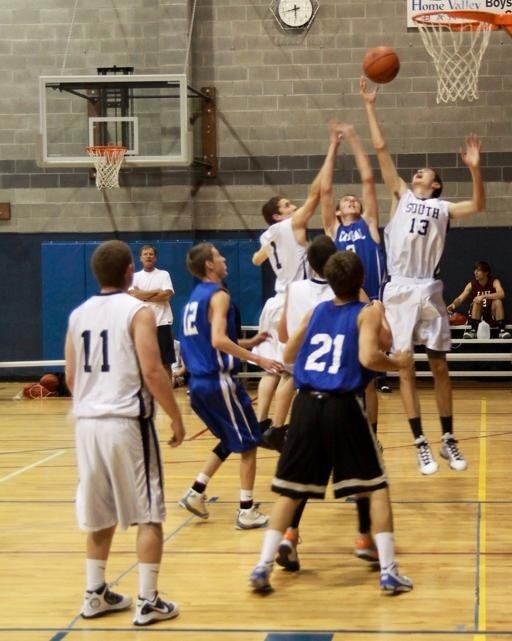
[130,244,175,386]
[446,262,510,338]
[63,241,186,630]
[358,74,484,477]
[247,251,414,595]
[246,120,385,458]
[277,235,395,572]
[175,245,284,529]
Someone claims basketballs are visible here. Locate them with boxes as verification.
[363,46,399,82]
[39,374,59,392]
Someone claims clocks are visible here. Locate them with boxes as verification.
[268,0,320,31]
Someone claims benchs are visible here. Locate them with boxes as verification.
[230,322,512,391]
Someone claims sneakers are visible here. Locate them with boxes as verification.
[262,424,289,452]
[132,590,178,626]
[498,329,511,339]
[354,531,379,562]
[258,418,271,449]
[235,501,303,594]
[439,432,467,471]
[379,559,413,593]
[79,583,133,618]
[463,329,476,339]
[178,488,210,519]
[413,435,438,475]
[377,382,391,392]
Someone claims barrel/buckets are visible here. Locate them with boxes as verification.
[477,320,491,339]
